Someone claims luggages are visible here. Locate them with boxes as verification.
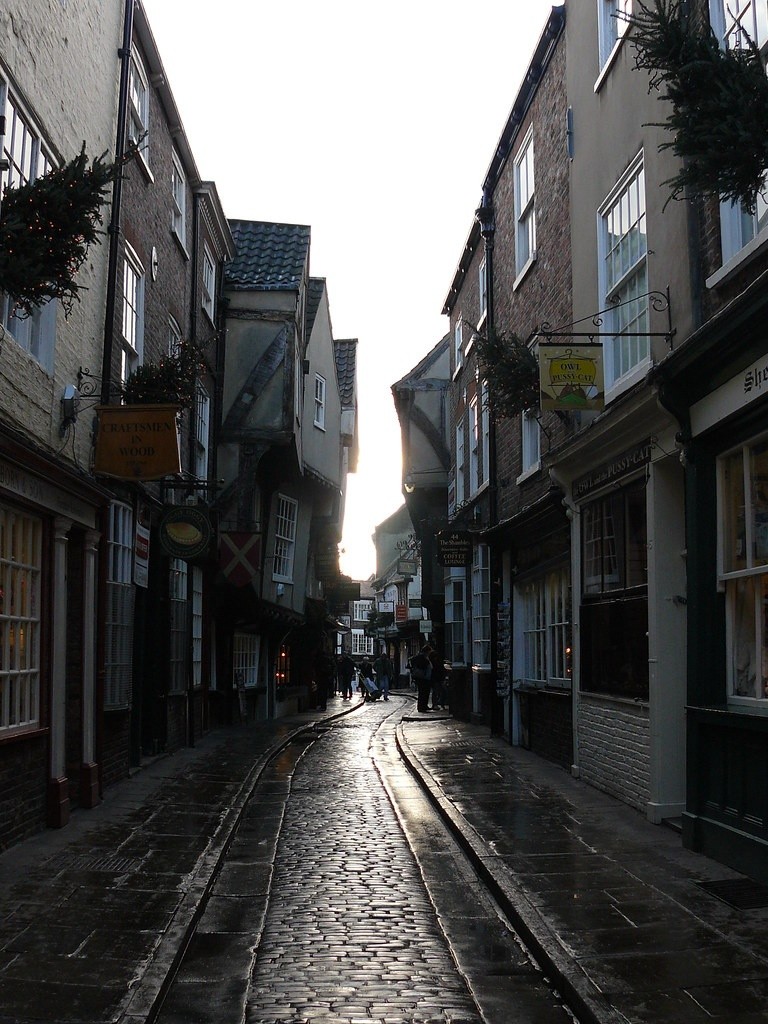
[357,670,381,702]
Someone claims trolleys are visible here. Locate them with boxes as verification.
[355,670,381,703]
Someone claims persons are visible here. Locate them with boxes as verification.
[313,650,355,711]
[358,656,372,697]
[372,652,392,701]
[412,645,446,712]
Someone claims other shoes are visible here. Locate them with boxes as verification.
[433,705,440,710]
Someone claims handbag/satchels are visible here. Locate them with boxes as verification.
[412,668,423,682]
